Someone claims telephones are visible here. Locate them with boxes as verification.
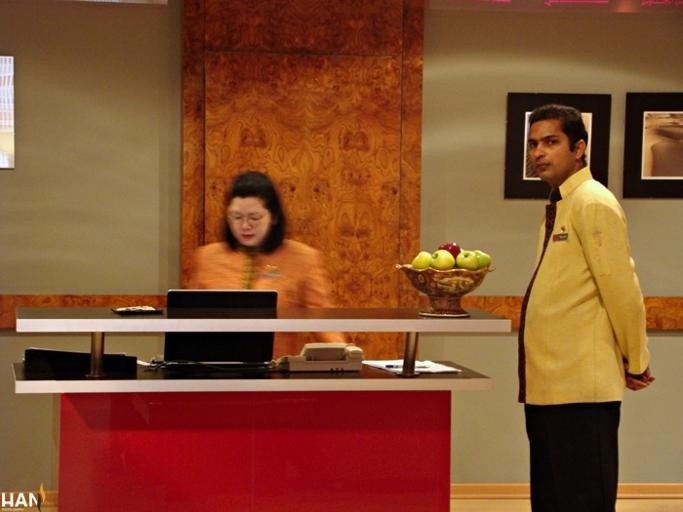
[276,343,364,372]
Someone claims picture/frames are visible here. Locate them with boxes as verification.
[503,92,613,200]
[621,91,683,200]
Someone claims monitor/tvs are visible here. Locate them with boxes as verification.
[163,289,278,363]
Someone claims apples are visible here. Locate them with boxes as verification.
[411,243,492,270]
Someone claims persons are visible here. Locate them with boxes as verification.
[518,106,655,512]
[189,170,346,360]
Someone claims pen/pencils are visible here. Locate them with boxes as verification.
[385,365,430,368]
